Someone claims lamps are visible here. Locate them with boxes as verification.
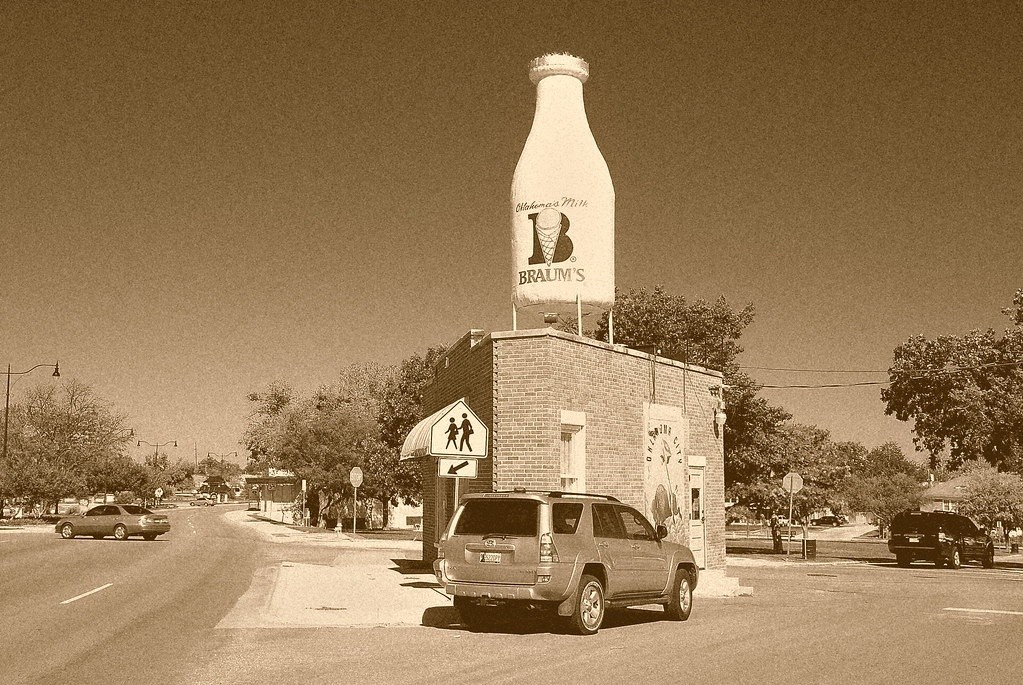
[715,400,726,426]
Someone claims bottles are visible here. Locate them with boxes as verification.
[509,53,613,306]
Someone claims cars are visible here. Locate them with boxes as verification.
[54,503,170,542]
[189,497,216,507]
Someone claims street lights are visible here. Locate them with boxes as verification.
[207,451,238,478]
[136,440,178,469]
[0,360,61,522]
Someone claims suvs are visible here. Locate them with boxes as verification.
[887,510,995,570]
[433,486,699,635]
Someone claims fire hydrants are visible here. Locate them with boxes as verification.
[336,522,344,539]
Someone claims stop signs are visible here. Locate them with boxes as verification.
[349,467,363,488]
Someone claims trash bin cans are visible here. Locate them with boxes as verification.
[1011,544,1018,555]
[801,538,817,560]
